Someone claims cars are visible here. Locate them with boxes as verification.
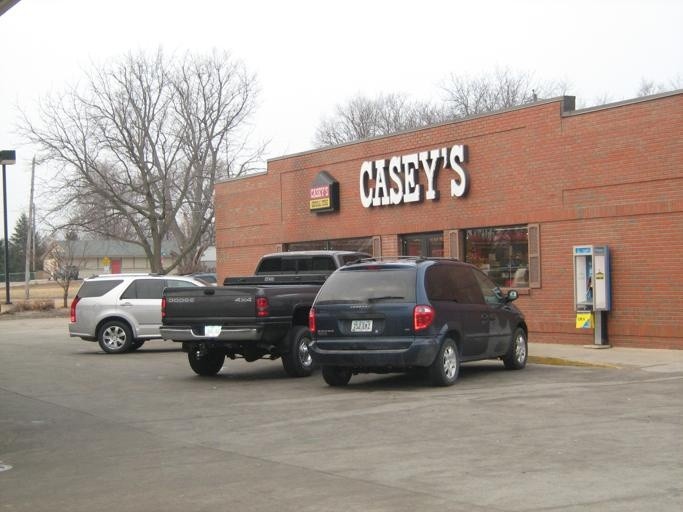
[308,256,527,387]
[60,265,79,280]
[69,272,217,354]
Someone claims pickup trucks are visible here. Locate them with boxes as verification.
[159,250,376,378]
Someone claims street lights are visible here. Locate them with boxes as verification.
[0,150,16,304]
